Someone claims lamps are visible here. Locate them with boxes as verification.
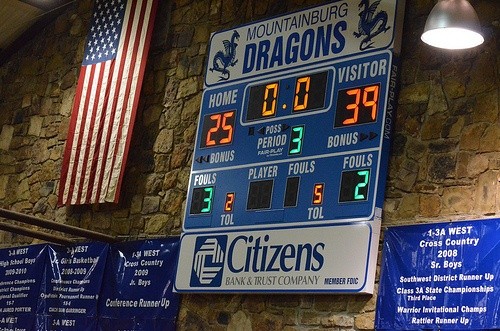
[419,0,487,50]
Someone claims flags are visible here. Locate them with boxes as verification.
[55,1,160,208]
